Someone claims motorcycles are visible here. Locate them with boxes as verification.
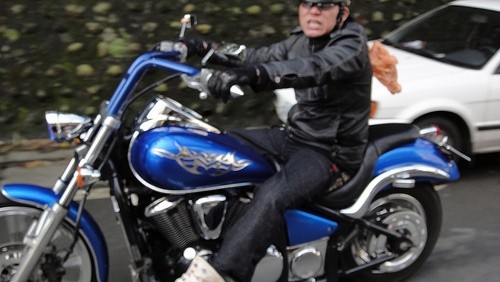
[0,13,472,282]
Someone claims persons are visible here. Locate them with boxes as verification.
[168,0,372,282]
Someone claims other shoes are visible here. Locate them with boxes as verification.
[172,257,227,282]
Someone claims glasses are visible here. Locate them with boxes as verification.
[301,1,340,9]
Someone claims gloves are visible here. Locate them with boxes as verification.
[186,39,205,57]
[208,67,249,104]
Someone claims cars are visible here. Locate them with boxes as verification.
[274,0,500,183]
